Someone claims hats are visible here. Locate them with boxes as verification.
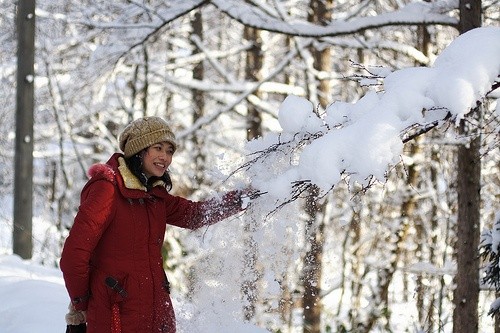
[119,116,178,159]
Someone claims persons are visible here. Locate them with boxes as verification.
[60,116,254,333]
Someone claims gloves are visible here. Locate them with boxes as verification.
[65,310,86,333]
